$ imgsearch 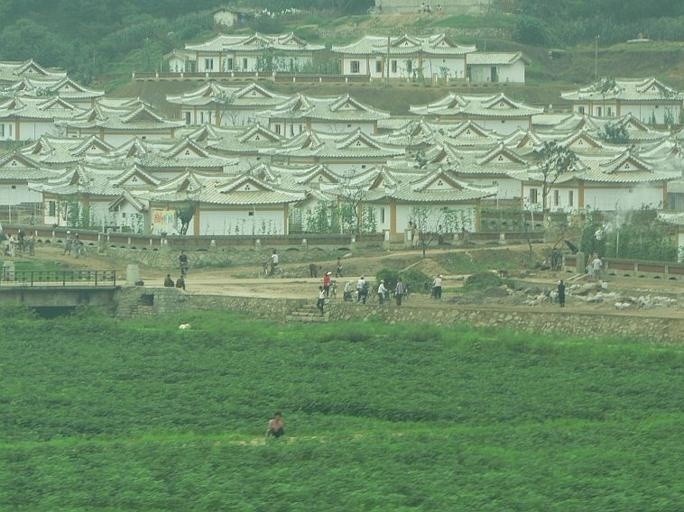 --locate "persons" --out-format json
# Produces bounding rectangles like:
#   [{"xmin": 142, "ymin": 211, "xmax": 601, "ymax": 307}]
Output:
[
  {"xmin": 264, "ymin": 250, "xmax": 279, "ymax": 276},
  {"xmin": 176, "ymin": 276, "xmax": 184, "ymax": 290},
  {"xmin": 62, "ymin": 230, "xmax": 73, "ymax": 256},
  {"xmin": 0, "ymin": 226, "xmax": 35, "ymax": 260},
  {"xmin": 163, "ymin": 273, "xmax": 173, "ymax": 288},
  {"xmin": 265, "ymin": 412, "xmax": 285, "ymax": 438},
  {"xmin": 437, "ymin": 225, "xmax": 444, "ymax": 244},
  {"xmin": 72, "ymin": 232, "xmax": 83, "ymax": 256},
  {"xmin": 592, "ymin": 255, "xmax": 603, "ymax": 279},
  {"xmin": 179, "ymin": 251, "xmax": 188, "ymax": 276},
  {"xmin": 557, "ymin": 280, "xmax": 567, "ymax": 308},
  {"xmin": 307, "ymin": 257, "xmax": 407, "ymax": 317},
  {"xmin": 432, "ymin": 274, "xmax": 442, "ymax": 298}
]
